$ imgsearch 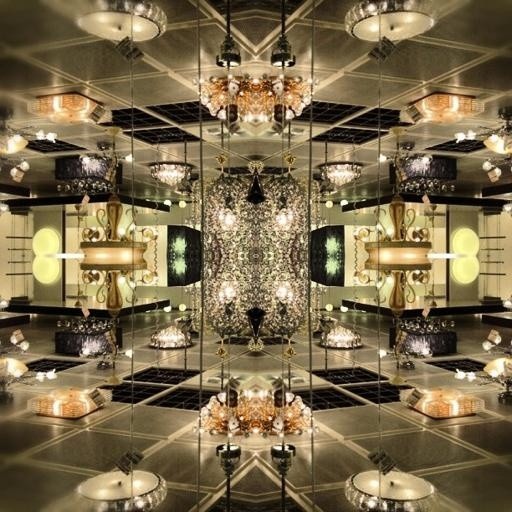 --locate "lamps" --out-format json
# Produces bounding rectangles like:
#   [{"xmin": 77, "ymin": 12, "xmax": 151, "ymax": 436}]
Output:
[{"xmin": 0, "ymin": 2, "xmax": 510, "ymax": 512}]
